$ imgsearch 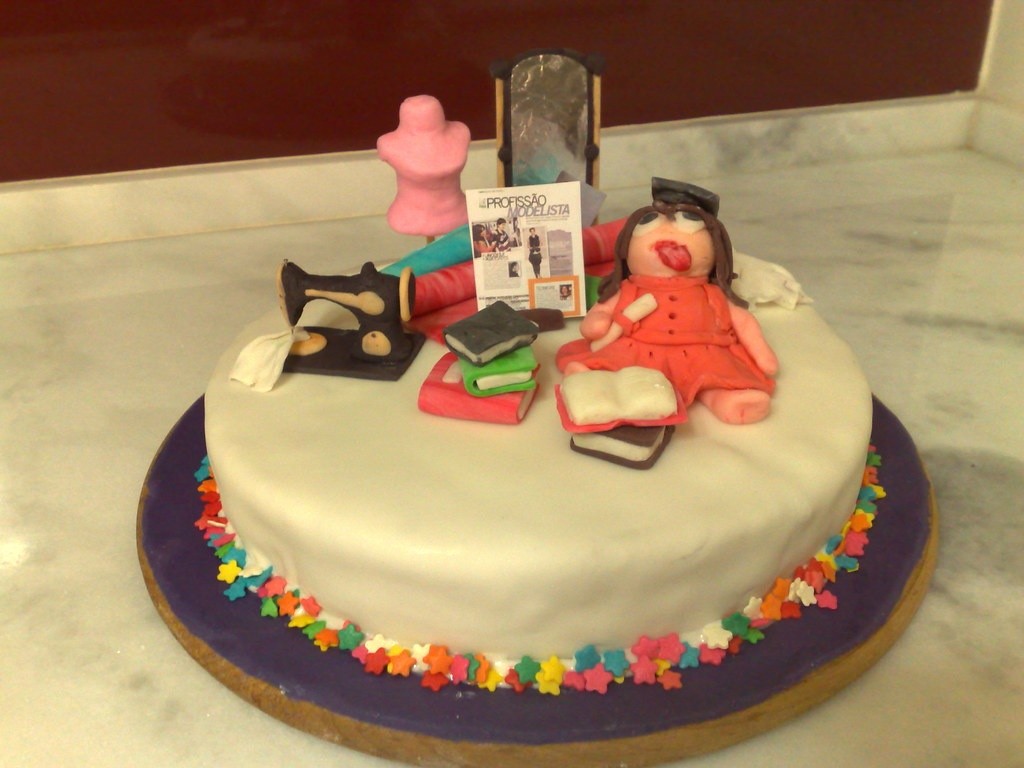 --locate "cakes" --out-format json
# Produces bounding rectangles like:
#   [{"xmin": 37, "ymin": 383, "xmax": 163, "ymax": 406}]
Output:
[{"xmin": 196, "ymin": 40, "xmax": 885, "ymax": 692}]
